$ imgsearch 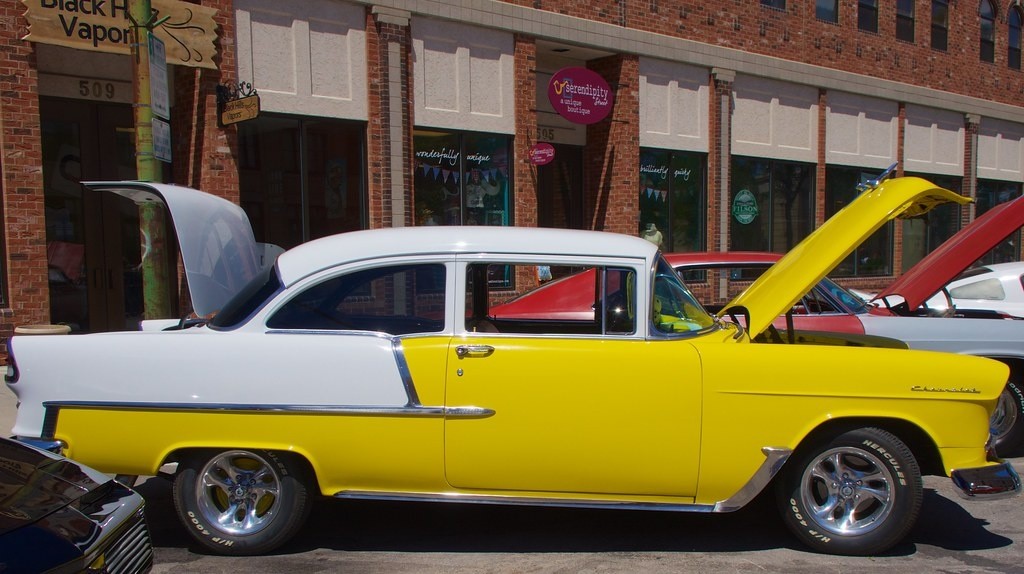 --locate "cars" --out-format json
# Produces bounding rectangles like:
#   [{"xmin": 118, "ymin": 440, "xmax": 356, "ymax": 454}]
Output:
[
  {"xmin": 0, "ymin": 435, "xmax": 154, "ymax": 574},
  {"xmin": 3, "ymin": 157, "xmax": 1013, "ymax": 561},
  {"xmin": 418, "ymin": 187, "xmax": 1023, "ymax": 462}
]
